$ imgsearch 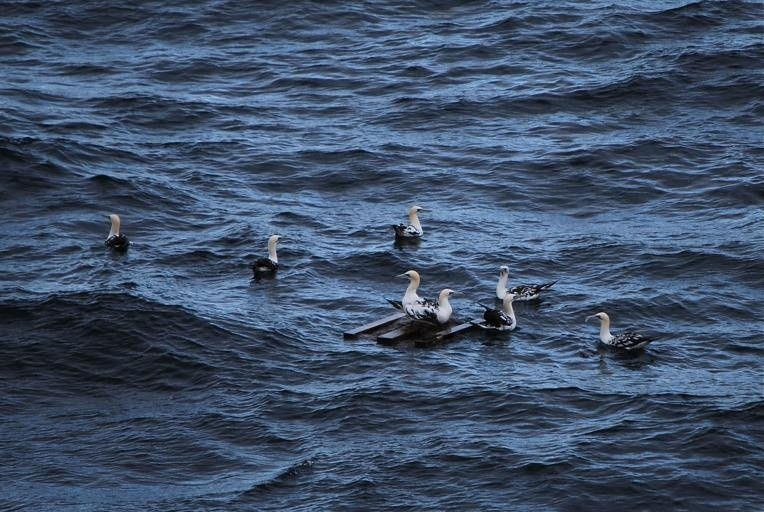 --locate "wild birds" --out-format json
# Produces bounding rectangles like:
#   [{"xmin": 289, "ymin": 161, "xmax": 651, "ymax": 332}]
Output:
[
  {"xmin": 385, "ymin": 288, "xmax": 465, "ymax": 325},
  {"xmin": 100, "ymin": 213, "xmax": 130, "ymax": 250},
  {"xmin": 391, "ymin": 269, "xmax": 421, "ymax": 305},
  {"xmin": 251, "ymin": 234, "xmax": 291, "ymax": 273},
  {"xmin": 390, "ymin": 205, "xmax": 432, "ymax": 238},
  {"xmin": 494, "ymin": 265, "xmax": 560, "ymax": 301},
  {"xmin": 469, "ymin": 293, "xmax": 526, "ymax": 332},
  {"xmin": 584, "ymin": 312, "xmax": 656, "ymax": 351}
]
